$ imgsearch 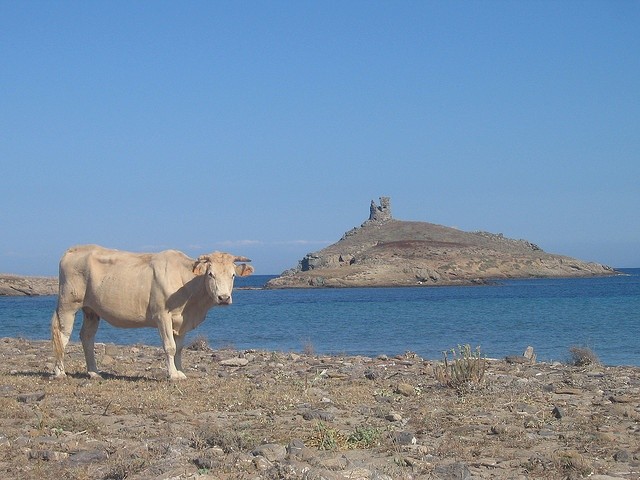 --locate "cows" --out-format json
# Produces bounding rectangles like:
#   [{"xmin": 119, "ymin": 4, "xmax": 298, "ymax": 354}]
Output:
[{"xmin": 51, "ymin": 244, "xmax": 254, "ymax": 382}]
